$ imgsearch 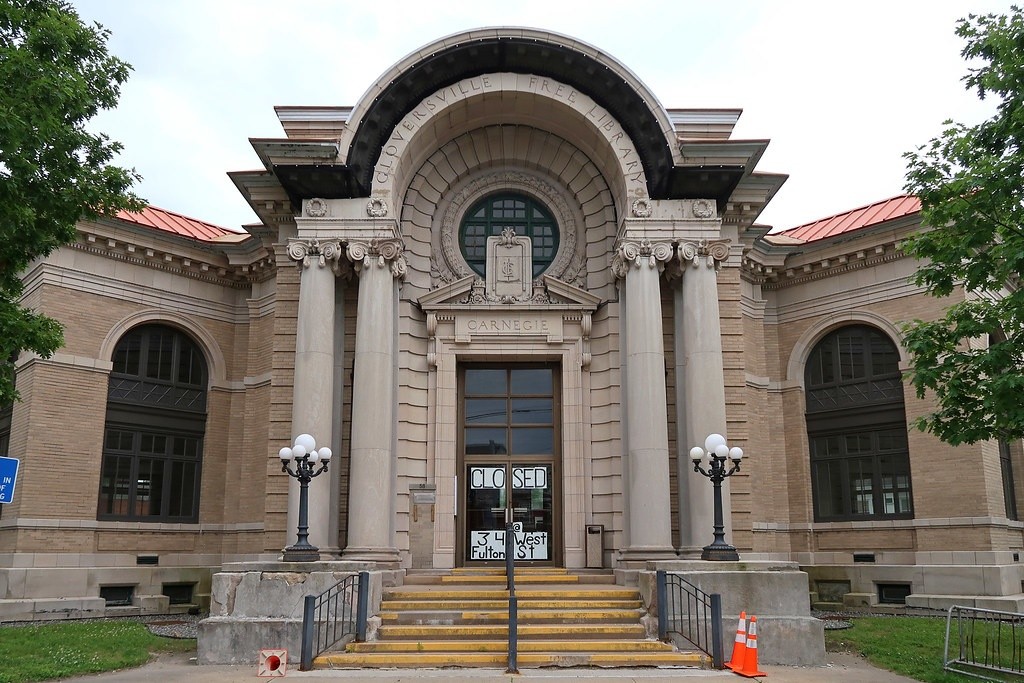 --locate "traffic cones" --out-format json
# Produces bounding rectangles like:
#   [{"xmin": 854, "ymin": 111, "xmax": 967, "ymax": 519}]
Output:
[
  {"xmin": 724, "ymin": 611, "xmax": 747, "ymax": 669},
  {"xmin": 733, "ymin": 615, "xmax": 768, "ymax": 677},
  {"xmin": 258, "ymin": 647, "xmax": 287, "ymax": 680}
]
